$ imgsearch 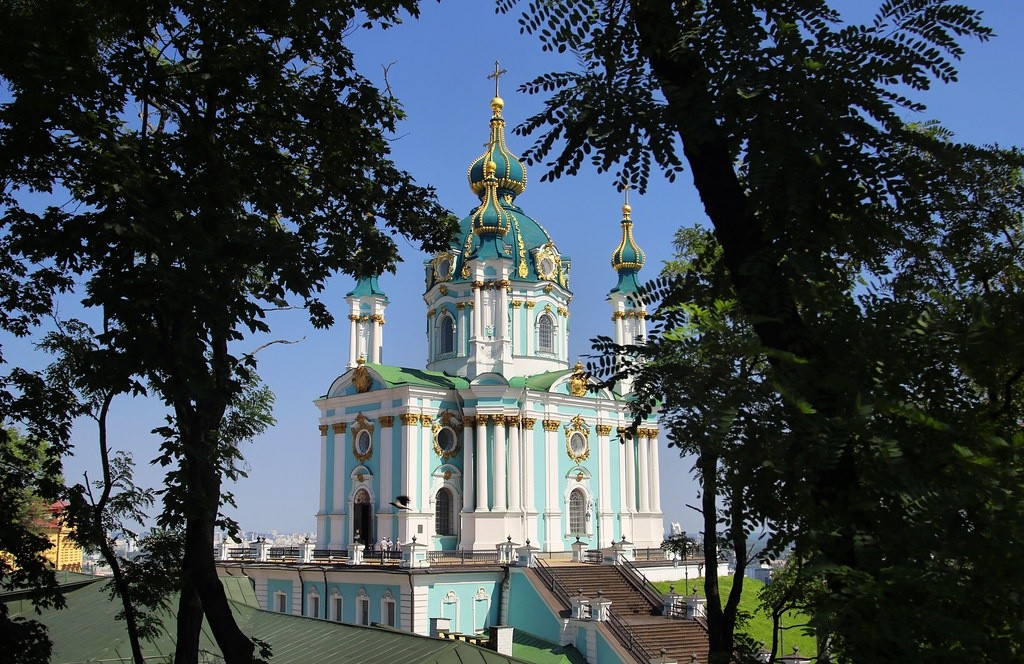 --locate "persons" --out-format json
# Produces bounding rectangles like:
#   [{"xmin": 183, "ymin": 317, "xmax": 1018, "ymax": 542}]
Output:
[
  {"xmin": 394, "ymin": 538, "xmax": 402, "ymax": 561},
  {"xmin": 386, "ymin": 538, "xmax": 393, "ymax": 559},
  {"xmin": 380, "ymin": 536, "xmax": 387, "ymax": 562}
]
